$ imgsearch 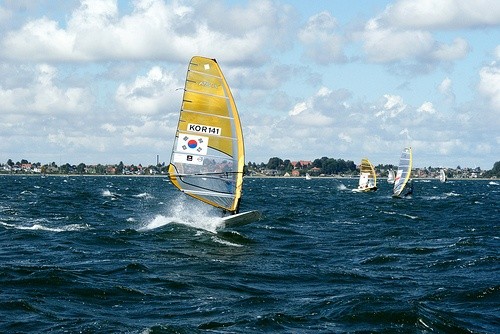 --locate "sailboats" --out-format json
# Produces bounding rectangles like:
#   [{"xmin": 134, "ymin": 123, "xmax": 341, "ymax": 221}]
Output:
[
  {"xmin": 439, "ymin": 168, "xmax": 446, "ymax": 183},
  {"xmin": 351, "ymin": 158, "xmax": 378, "ymax": 192},
  {"xmin": 134, "ymin": 55, "xmax": 262, "ymax": 231},
  {"xmin": 393, "ymin": 145, "xmax": 414, "ymax": 198},
  {"xmin": 387, "ymin": 170, "xmax": 397, "ymax": 184}
]
